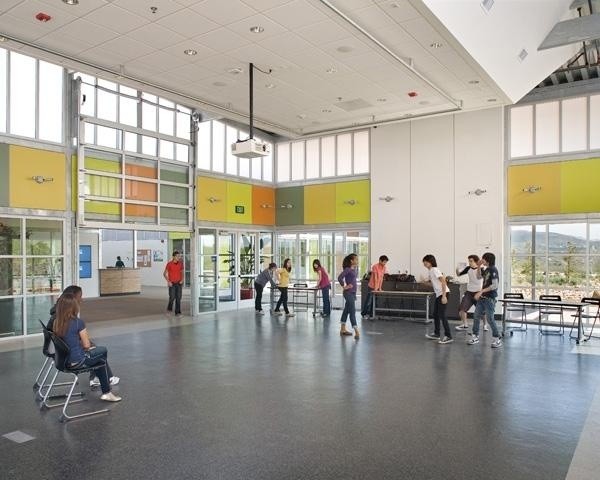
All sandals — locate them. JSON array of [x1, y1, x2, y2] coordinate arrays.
[[339, 330, 352, 335], [355, 332, 360, 340]]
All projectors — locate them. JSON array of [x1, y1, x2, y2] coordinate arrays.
[[232, 139, 270, 159]]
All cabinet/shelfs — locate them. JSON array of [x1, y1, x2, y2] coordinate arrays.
[[97, 266, 142, 296], [358, 277, 468, 322]]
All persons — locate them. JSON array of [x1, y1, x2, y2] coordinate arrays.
[[253, 261, 276, 315], [162, 251, 185, 320], [466, 251, 505, 348], [114, 256, 125, 267], [422, 255, 454, 344], [337, 253, 361, 337], [53, 292, 125, 403], [453, 253, 490, 333], [360, 254, 389, 322], [45, 285, 100, 387], [272, 258, 296, 317], [311, 260, 332, 317]]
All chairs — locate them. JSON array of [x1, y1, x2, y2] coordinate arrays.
[[394, 279, 417, 322], [537, 293, 565, 336], [569, 293, 600, 344], [291, 282, 312, 313], [38, 330, 112, 423], [28, 317, 87, 399], [499, 290, 530, 333]]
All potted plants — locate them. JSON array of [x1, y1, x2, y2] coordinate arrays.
[[219, 235, 272, 299]]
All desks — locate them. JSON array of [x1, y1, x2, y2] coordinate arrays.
[[497, 296, 590, 346], [266, 282, 332, 318], [370, 288, 439, 329]]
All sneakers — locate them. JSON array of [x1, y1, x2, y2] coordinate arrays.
[[425, 334, 440, 340], [490, 338, 502, 348], [164, 310, 171, 317], [259, 310, 265, 314], [285, 313, 296, 317], [108, 376, 121, 386], [483, 325, 489, 332], [466, 336, 480, 345], [438, 335, 454, 344], [454, 323, 469, 331], [361, 311, 369, 320], [174, 314, 184, 321], [100, 392, 122, 403], [369, 316, 377, 320], [88, 376, 100, 387], [256, 309, 259, 313], [274, 311, 282, 314]]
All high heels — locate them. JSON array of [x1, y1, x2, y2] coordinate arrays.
[[322, 312, 330, 318]]
